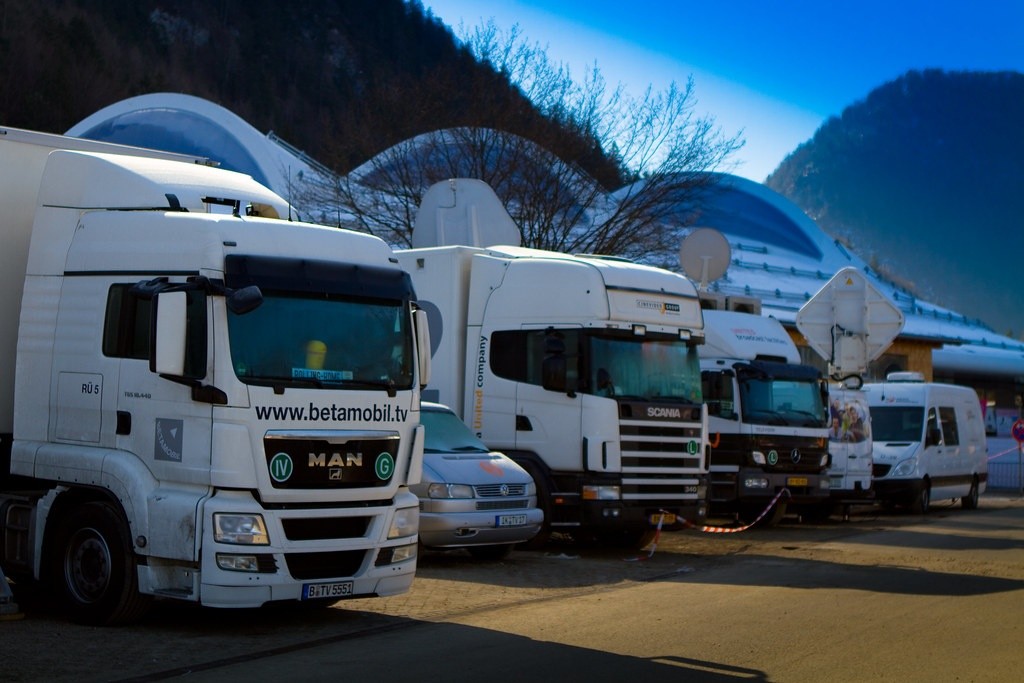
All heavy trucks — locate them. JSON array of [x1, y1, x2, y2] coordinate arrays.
[[1, 126, 431, 622], [393, 244, 721, 560]]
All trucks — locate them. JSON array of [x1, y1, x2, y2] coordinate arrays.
[[643, 310, 836, 531]]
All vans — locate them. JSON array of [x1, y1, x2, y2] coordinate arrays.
[[861, 372, 989, 516], [773, 379, 875, 525]]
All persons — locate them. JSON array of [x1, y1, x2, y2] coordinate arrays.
[[829, 396, 869, 444]]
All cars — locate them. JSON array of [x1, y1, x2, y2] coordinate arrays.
[[412, 398, 545, 553]]
